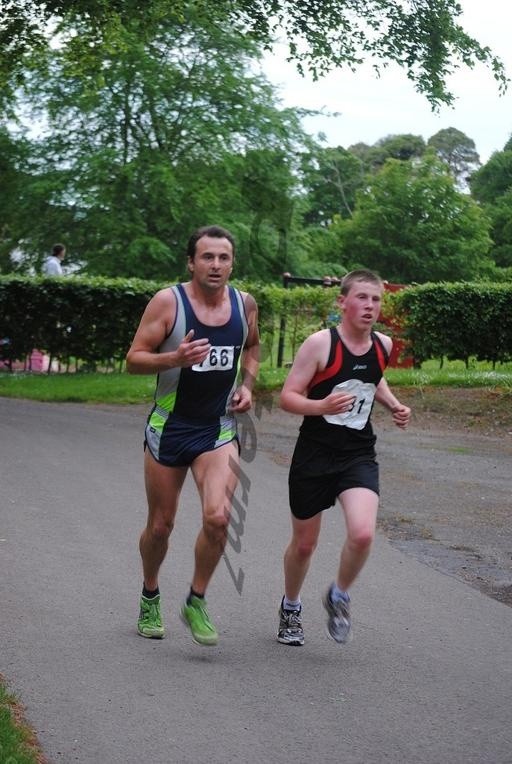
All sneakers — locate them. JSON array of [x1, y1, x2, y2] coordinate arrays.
[[182, 591, 217, 645], [138, 593, 164, 639], [279, 593, 305, 648], [323, 586, 350, 641]]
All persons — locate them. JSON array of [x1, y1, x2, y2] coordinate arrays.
[[40, 244, 65, 275], [278, 270, 412, 646], [125, 225, 260, 645]]
